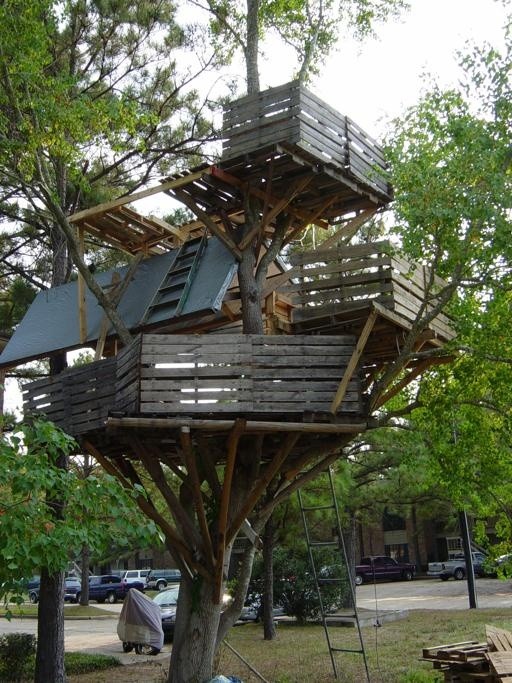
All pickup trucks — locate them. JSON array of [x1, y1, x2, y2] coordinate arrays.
[[355, 556, 417, 585], [427, 552, 488, 581]]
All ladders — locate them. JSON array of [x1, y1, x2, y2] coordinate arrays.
[[295, 465, 370, 683]]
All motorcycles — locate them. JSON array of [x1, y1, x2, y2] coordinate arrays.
[[121, 642, 160, 654]]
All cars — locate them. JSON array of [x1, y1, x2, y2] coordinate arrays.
[[28, 564, 283, 637], [485, 553, 512, 579]]
